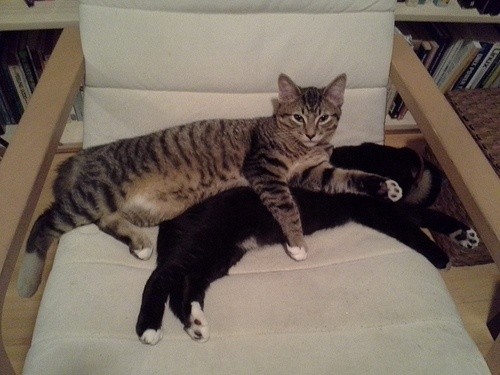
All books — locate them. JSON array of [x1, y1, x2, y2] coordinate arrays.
[[397, 0, 500, 17], [388, 28, 500, 126], [0, 32, 86, 133]]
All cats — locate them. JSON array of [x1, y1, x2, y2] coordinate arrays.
[[14, 71, 402, 299], [135, 141, 479, 346]]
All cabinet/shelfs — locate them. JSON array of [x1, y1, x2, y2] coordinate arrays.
[[0, 14, 500, 153]]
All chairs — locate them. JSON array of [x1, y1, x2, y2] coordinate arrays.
[[0, 0, 500, 374]]
